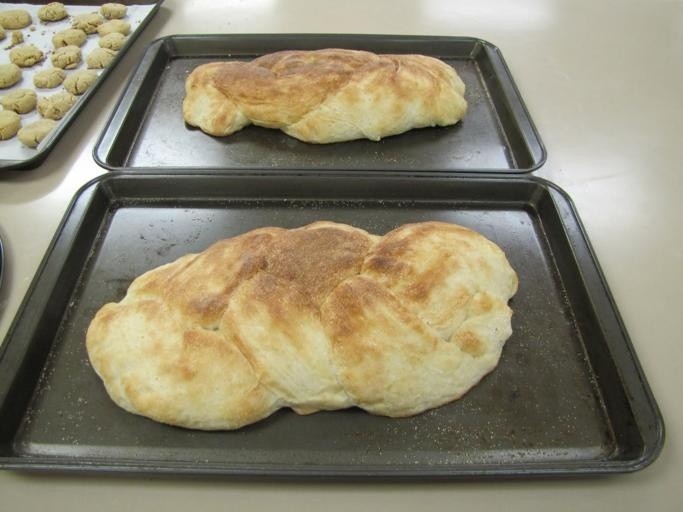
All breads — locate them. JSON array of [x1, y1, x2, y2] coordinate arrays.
[[84, 220, 518, 430], [182, 47, 469, 146]]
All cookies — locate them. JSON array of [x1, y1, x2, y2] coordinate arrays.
[[1, 2, 131, 148]]
